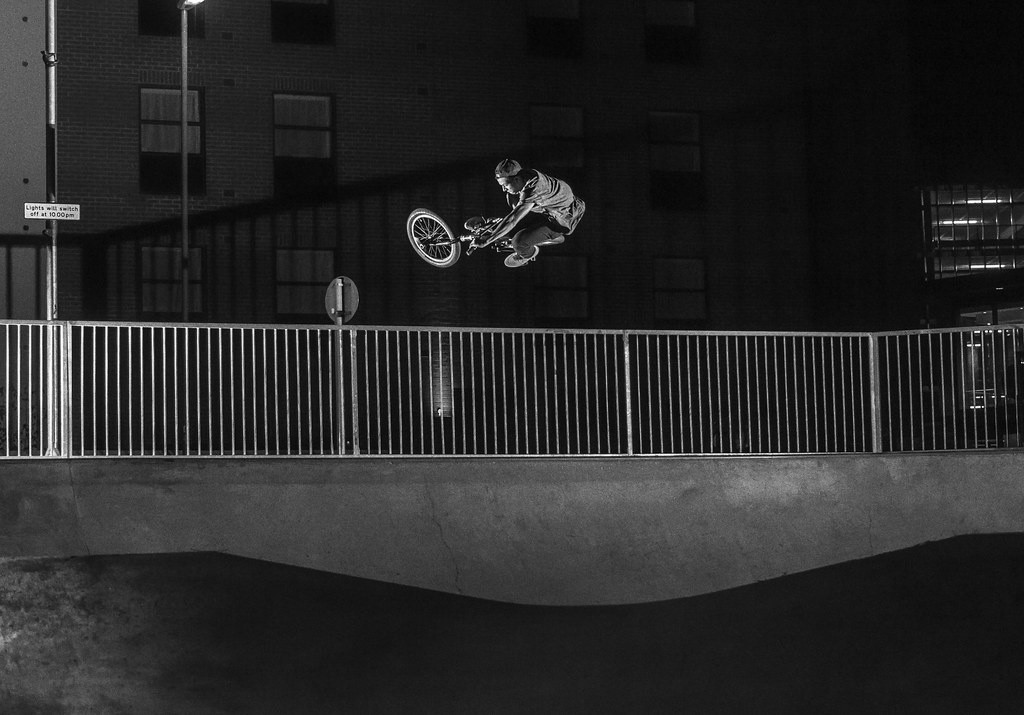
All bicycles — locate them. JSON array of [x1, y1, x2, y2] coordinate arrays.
[[405, 210, 566, 268]]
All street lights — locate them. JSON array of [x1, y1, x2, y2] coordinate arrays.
[[176, 0, 203, 320]]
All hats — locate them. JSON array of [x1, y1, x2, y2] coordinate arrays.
[[494, 158, 521, 177]]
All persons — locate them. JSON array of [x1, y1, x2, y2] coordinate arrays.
[[461, 159, 586, 272]]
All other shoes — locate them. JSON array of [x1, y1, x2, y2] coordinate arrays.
[[504, 244, 539, 268]]
